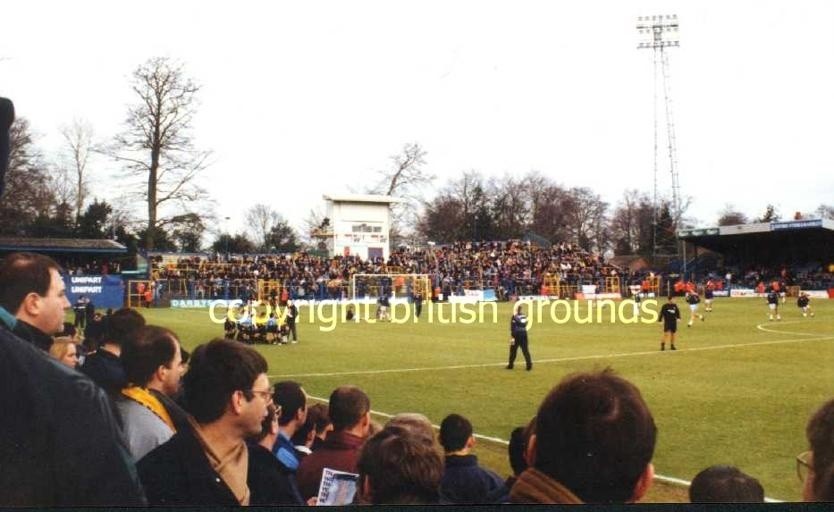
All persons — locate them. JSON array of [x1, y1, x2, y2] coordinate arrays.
[[0, 235, 833, 507]]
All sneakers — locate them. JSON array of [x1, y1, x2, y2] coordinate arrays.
[[661, 342, 677, 352]]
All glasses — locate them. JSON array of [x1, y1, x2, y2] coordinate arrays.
[[793, 447, 814, 480], [252, 384, 275, 401]]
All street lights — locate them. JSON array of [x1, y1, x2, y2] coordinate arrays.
[[637, 9, 685, 268]]
